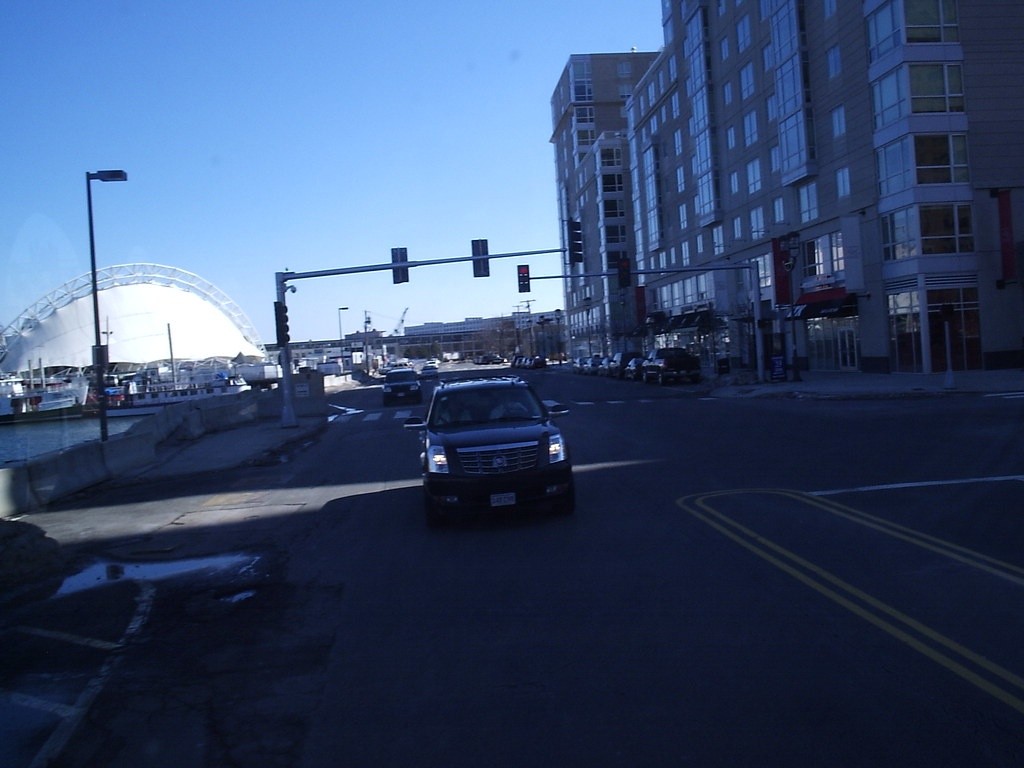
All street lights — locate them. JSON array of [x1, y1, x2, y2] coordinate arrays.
[[83, 169, 129, 440], [338, 306, 349, 374]]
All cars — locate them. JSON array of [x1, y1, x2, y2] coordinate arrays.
[[597, 355, 612, 377], [511, 354, 546, 369], [623, 356, 643, 380], [424, 360, 439, 370], [379, 369, 424, 406], [582, 357, 604, 376], [572, 356, 587, 375]]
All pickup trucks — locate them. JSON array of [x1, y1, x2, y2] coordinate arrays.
[[640, 347, 701, 386]]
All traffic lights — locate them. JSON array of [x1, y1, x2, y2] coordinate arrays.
[[567, 216, 584, 265], [516, 264, 531, 294], [616, 257, 632, 287], [274, 301, 290, 347]]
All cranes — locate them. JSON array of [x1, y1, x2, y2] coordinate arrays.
[[390, 307, 411, 337]]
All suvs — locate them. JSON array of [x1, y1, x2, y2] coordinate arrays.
[[609, 352, 638, 379], [401, 374, 578, 527]]
